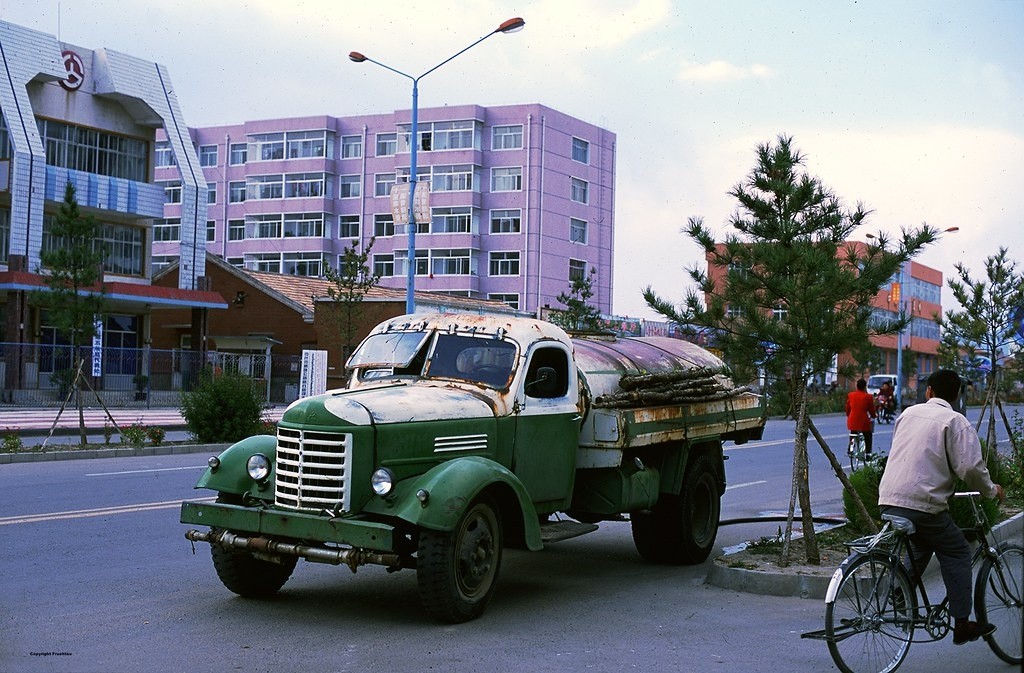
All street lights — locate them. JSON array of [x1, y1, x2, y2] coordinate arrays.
[[348, 18, 526, 310], [865, 226, 960, 415]]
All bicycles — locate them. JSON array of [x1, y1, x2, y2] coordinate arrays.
[[847, 415, 876, 472], [801, 488, 1023, 673]]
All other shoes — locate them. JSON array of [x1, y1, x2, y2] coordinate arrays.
[[883, 415, 886, 420], [866, 455, 872, 461], [890, 411, 896, 415], [889, 592, 925, 624], [952, 621, 997, 645], [847, 452, 854, 457]]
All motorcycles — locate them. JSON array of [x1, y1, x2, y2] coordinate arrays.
[[872, 393, 895, 425]]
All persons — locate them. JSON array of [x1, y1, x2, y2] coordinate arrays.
[[846, 378, 879, 461], [809, 379, 819, 394], [877, 380, 897, 420], [878, 369, 1005, 644]]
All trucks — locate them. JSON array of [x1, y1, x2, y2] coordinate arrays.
[[179, 303, 768, 625], [866, 374, 911, 407]]
